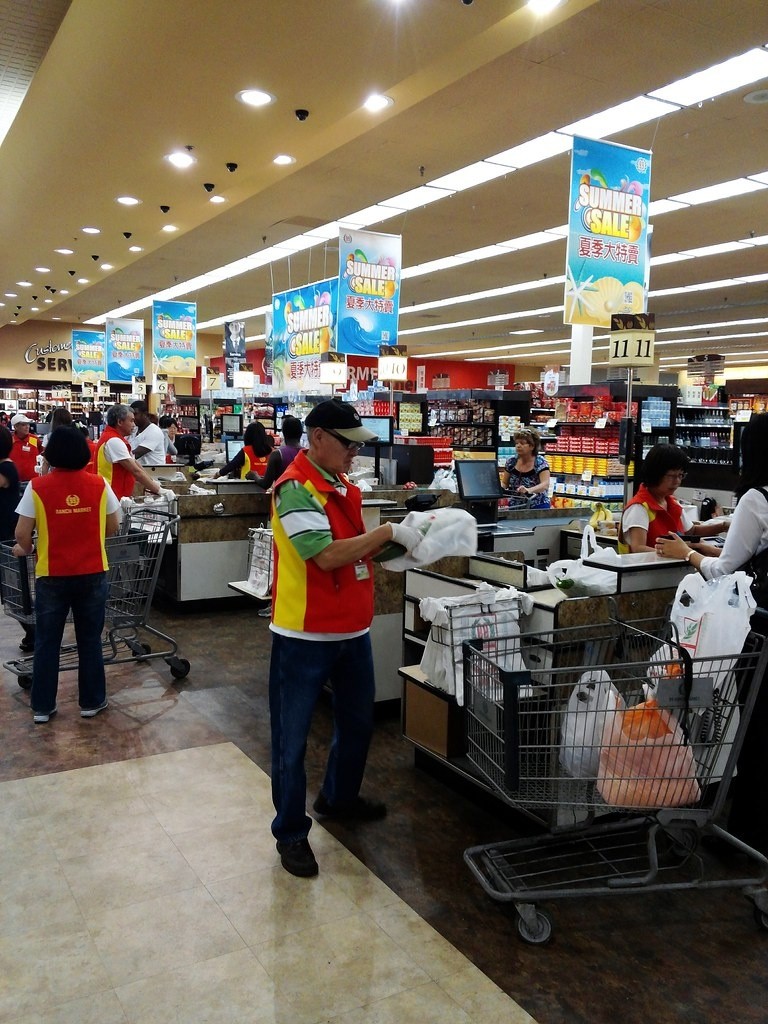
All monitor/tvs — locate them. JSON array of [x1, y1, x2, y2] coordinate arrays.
[[178, 415, 198, 432], [225, 439, 246, 463], [358, 416, 394, 447], [455, 459, 503, 499], [87, 426, 95, 441], [220, 414, 243, 435], [175, 434, 201, 455], [36, 423, 51, 434]]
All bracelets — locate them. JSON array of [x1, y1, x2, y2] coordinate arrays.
[[723, 521, 729, 533]]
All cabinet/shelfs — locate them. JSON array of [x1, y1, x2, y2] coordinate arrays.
[[531, 383, 638, 514], [38, 387, 71, 433], [0, 387, 38, 427], [642, 387, 739, 470], [71, 384, 146, 426], [423, 392, 497, 462], [157, 396, 200, 446]]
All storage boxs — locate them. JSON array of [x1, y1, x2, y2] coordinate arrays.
[[398, 666, 459, 759]]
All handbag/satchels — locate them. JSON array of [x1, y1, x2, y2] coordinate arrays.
[[559, 672, 628, 780], [596, 704, 701, 806], [735, 548, 768, 613], [650, 572, 756, 715]]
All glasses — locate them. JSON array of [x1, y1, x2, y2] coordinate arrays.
[[518, 428, 531, 432], [321, 427, 363, 450]]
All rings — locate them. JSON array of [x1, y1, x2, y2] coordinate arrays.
[[660, 550, 663, 554]]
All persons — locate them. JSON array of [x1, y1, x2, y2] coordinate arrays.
[[655, 412, 768, 870], [0, 401, 306, 617], [501, 426, 551, 510], [12, 424, 121, 722], [268, 397, 426, 877], [618, 443, 731, 555]]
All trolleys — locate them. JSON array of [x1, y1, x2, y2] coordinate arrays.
[[461, 596, 767, 945], [498, 489, 538, 510], [0, 507, 190, 689]]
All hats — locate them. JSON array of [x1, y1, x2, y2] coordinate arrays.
[[304, 398, 378, 443], [11, 414, 35, 425]]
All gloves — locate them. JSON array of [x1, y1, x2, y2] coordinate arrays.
[[387, 521, 425, 553]]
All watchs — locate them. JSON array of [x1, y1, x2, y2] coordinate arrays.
[[685, 550, 696, 562]]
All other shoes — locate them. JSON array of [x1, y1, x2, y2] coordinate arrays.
[[258, 603, 272, 617], [34, 704, 58, 721], [81, 698, 108, 716]]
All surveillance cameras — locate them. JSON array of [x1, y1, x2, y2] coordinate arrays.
[[16, 306, 22, 309], [44, 286, 51, 290], [92, 255, 99, 261], [123, 232, 132, 239], [32, 296, 38, 300], [295, 109, 309, 121], [68, 271, 75, 276], [50, 289, 56, 294], [160, 206, 170, 214], [226, 163, 238, 173], [203, 183, 215, 193], [14, 313, 19, 316]]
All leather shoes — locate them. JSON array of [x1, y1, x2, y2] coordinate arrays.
[[313, 790, 386, 819], [276, 838, 318, 877]]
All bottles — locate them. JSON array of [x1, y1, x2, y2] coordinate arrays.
[[731, 401, 749, 411], [675, 430, 730, 446], [71, 403, 83, 413], [276, 405, 288, 412], [675, 410, 733, 424], [643, 445, 654, 460], [643, 436, 657, 444], [41, 413, 45, 419], [88, 423, 94, 440], [658, 436, 669, 445]]
